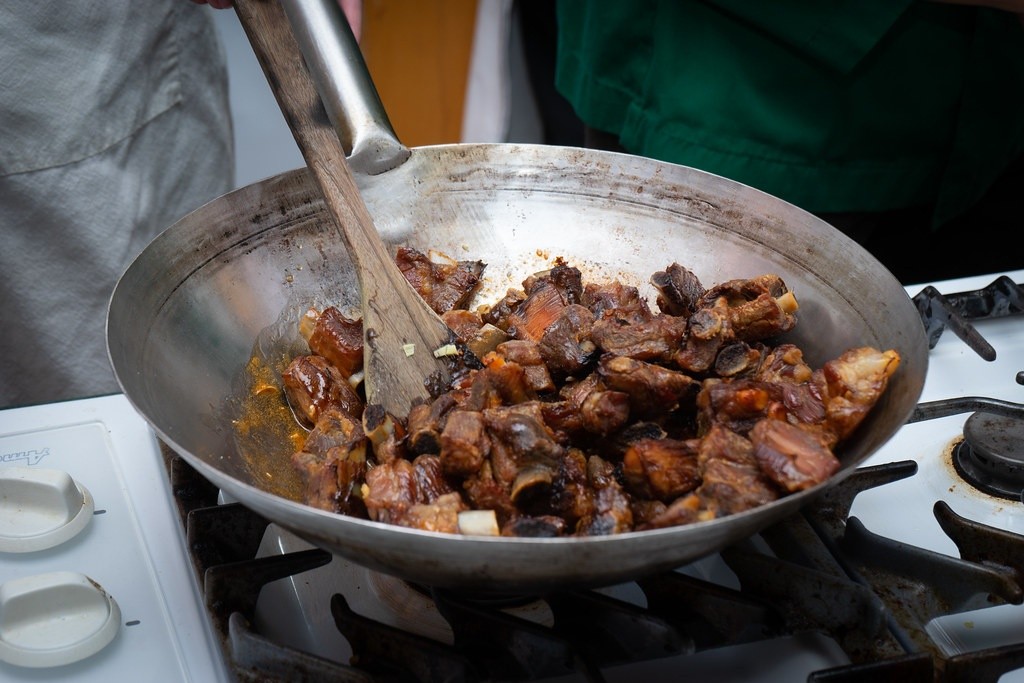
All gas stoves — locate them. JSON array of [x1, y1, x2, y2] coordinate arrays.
[[2, 263, 1024, 683]]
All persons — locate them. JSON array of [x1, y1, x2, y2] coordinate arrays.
[[0, 1, 366, 410], [555, 3, 1024, 294]]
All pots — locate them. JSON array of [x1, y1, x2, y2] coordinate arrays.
[[105, 1, 931, 595]]
